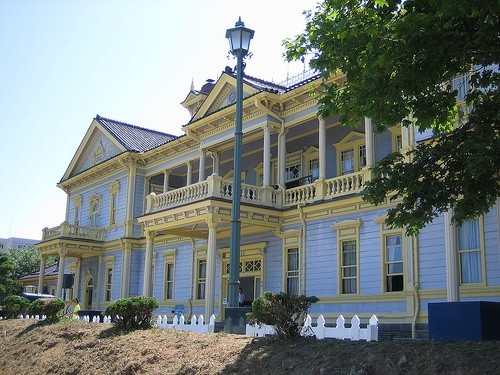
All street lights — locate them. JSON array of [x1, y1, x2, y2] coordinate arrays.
[[224, 15, 257, 308]]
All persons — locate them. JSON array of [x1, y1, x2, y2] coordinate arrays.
[[239, 287, 246, 307], [72, 297, 81, 319]]
